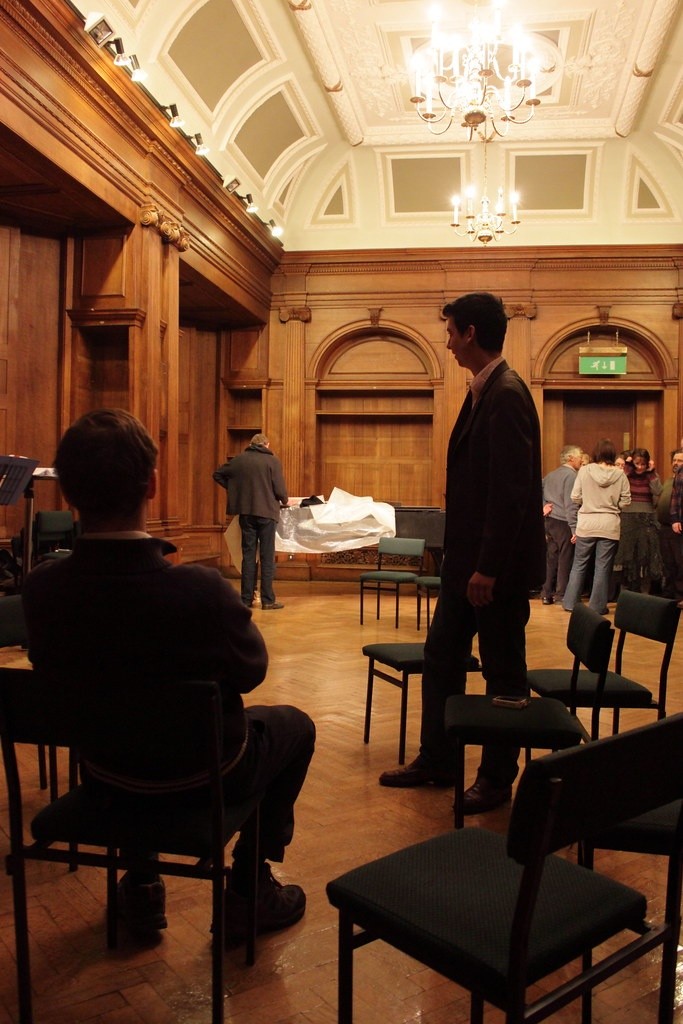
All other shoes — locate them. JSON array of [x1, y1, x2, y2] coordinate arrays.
[[262, 602, 284, 610], [542, 595, 563, 605], [602, 608, 609, 615]]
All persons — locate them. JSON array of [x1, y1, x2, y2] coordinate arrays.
[[380, 291, 545, 814], [23, 410, 316, 940], [212, 434, 296, 609], [541, 439, 683, 615]]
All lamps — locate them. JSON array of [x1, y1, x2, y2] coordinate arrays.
[[128, 54, 148, 82], [162, 103, 186, 128], [410, 0, 542, 144], [188, 132, 211, 155], [222, 173, 242, 195], [106, 36, 131, 67], [240, 193, 259, 215], [262, 219, 284, 236], [84, 12, 115, 48], [449, 117, 522, 246]]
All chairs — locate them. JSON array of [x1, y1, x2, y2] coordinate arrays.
[[576, 798, 683, 872], [525, 588, 683, 761], [357, 537, 425, 629], [361, 639, 484, 764], [328, 710, 683, 1024], [0, 664, 271, 1024], [443, 602, 616, 829], [414, 575, 441, 632]]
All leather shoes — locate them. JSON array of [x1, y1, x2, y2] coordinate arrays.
[[117, 873, 167, 935], [379, 753, 458, 789], [458, 780, 513, 813], [209, 862, 305, 940]]
